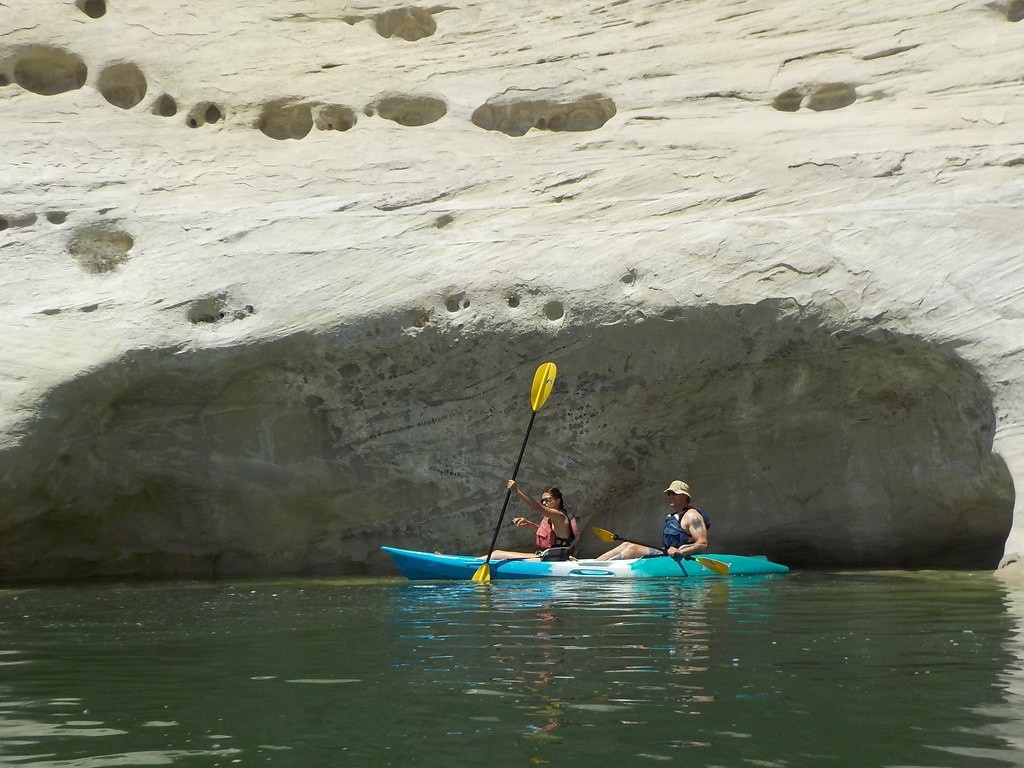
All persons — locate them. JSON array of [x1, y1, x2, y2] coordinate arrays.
[[569, 481, 709, 561], [435, 480, 575, 563]]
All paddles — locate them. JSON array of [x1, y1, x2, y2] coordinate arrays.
[[592, 525, 729, 577], [470, 362, 558, 581]]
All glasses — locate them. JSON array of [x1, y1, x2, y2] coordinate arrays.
[[666, 491, 678, 496], [540, 497, 559, 505]]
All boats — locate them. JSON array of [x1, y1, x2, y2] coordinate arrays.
[[379, 536, 791, 582]]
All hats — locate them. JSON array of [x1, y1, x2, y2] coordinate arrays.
[[663, 480, 693, 499]]
